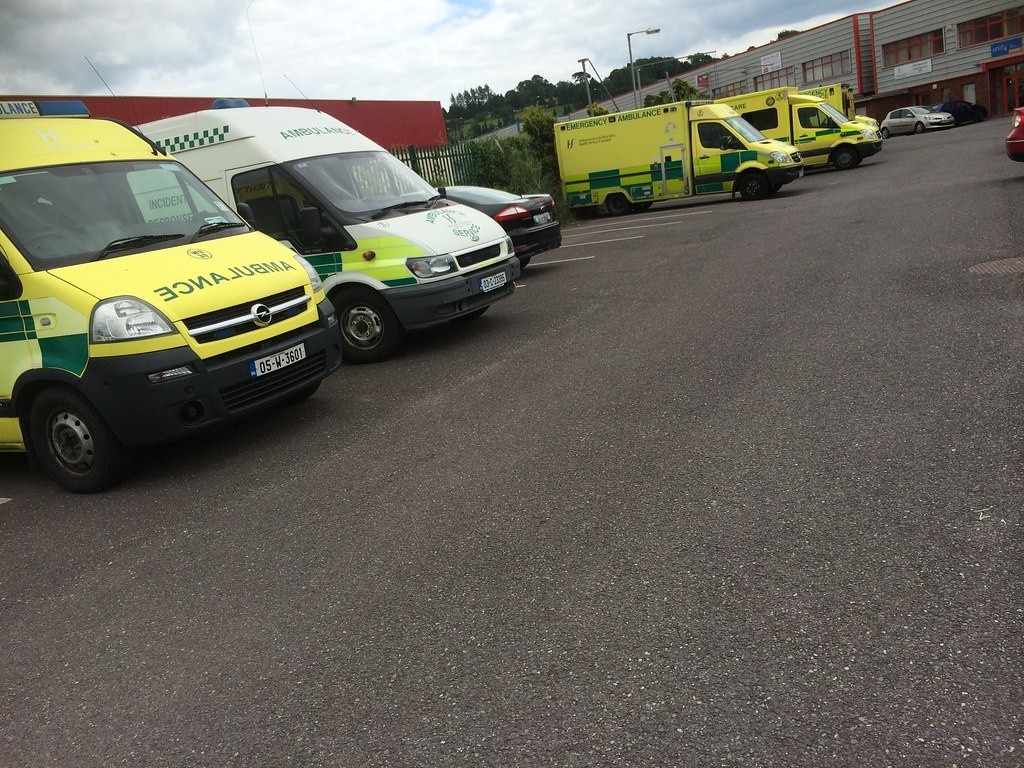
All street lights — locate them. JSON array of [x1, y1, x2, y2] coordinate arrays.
[[577, 57, 597, 117], [626, 28, 661, 109]]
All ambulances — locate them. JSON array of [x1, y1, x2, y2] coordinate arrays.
[[707, 86, 883, 170], [0, 101, 344, 497], [124, 99, 522, 362], [797, 83, 880, 130], [553, 100, 805, 215]]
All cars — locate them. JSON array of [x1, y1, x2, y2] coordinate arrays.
[[879, 106, 955, 138], [1005, 107, 1024, 162], [394, 185, 563, 270], [931, 99, 989, 126]]
